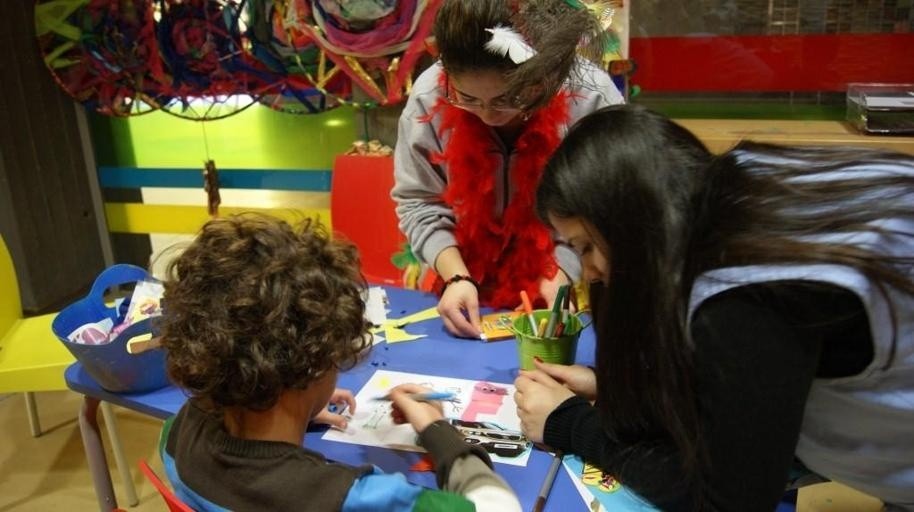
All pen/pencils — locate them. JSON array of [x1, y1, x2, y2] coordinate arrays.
[[531, 448, 564, 512], [520, 285, 571, 338], [373, 392, 452, 401]]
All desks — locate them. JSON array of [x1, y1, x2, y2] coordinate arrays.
[[669, 114, 914, 169], [62, 280, 798, 512]]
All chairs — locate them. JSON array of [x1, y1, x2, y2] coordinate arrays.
[[134, 459, 191, 512], [0, 235, 143, 511]]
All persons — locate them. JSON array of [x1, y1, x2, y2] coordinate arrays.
[[513, 103, 914, 511], [388, 0, 631, 342], [155, 206, 525, 512]]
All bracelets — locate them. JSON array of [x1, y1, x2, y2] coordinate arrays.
[[438, 272, 483, 293]]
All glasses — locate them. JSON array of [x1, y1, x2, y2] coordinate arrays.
[[444, 70, 525, 114]]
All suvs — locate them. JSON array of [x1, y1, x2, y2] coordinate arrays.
[[417, 420, 532, 460]]
